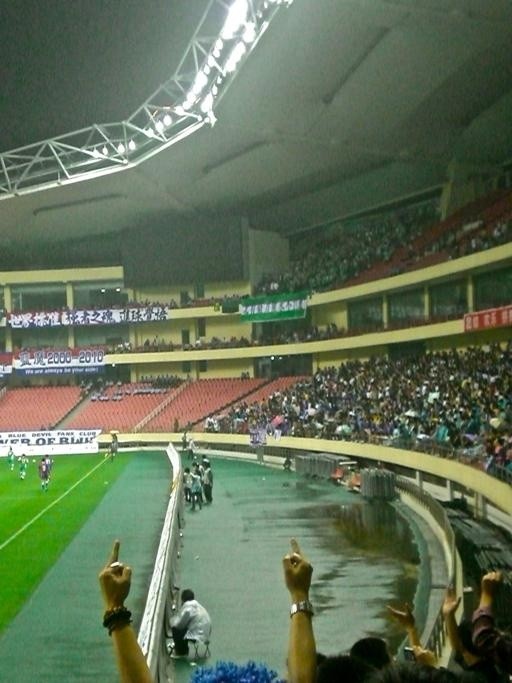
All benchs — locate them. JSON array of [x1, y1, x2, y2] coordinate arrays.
[[419, 486, 512, 682]]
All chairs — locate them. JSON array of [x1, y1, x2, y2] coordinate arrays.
[[330, 181, 512, 292], [0, 375, 313, 437]]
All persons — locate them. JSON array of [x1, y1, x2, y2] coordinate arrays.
[[0, 181, 512, 682]]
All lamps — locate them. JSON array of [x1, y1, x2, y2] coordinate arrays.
[[81, 110, 182, 159]]
